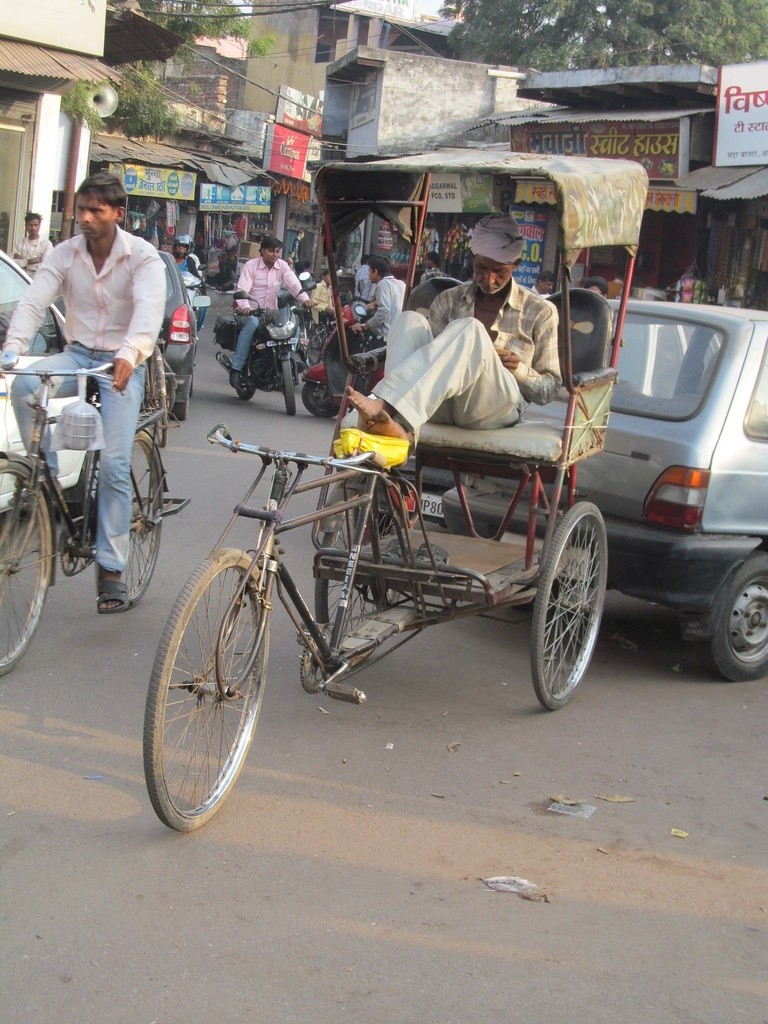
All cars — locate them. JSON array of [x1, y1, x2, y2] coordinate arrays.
[[0, 249, 88, 514], [53, 250, 211, 421]]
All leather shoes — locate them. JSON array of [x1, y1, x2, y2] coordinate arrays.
[[230, 368, 242, 390]]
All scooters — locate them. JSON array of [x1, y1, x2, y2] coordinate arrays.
[[180, 265, 208, 320], [300, 305, 384, 418]]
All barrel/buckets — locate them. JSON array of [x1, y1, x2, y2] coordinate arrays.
[[62, 413, 96, 450]]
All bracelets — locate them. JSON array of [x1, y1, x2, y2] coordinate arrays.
[[361, 323, 365, 331]]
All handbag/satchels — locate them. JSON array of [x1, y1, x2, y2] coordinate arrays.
[[51, 369, 105, 450]]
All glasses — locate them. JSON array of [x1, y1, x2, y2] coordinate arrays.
[[177, 243, 187, 248]]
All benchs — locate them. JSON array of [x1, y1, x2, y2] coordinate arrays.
[[339, 276, 611, 465]]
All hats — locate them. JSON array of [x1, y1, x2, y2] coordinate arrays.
[[468, 213, 524, 262]]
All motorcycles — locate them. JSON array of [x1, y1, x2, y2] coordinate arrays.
[[212, 281, 317, 415]]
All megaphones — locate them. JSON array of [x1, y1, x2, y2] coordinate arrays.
[[88, 85, 119, 117]]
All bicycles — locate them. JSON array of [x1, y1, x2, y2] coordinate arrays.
[[305, 312, 335, 365], [0, 348, 192, 676]]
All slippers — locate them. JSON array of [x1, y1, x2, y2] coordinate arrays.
[[8, 491, 30, 512], [385, 538, 409, 558], [97, 576, 129, 614], [416, 543, 449, 567]]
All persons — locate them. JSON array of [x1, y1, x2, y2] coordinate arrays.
[[420, 252, 451, 285], [13, 213, 54, 280], [173, 233, 207, 341], [531, 271, 556, 298], [345, 212, 563, 468], [583, 276, 608, 295], [132, 228, 144, 238], [229, 237, 317, 389], [286, 250, 295, 273], [293, 230, 304, 276], [355, 255, 374, 317], [351, 256, 407, 393], [310, 268, 335, 317], [0, 170, 167, 614]]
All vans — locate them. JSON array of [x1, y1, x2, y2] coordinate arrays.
[[443, 299, 768, 682]]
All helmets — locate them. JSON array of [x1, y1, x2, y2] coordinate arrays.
[[173, 236, 190, 256], [182, 234, 195, 252]]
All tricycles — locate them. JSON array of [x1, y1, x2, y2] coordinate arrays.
[[142, 149, 648, 833]]
